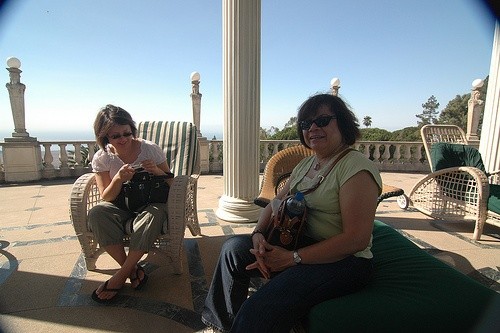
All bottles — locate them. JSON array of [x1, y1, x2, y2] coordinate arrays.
[[122, 178, 135, 193], [285, 191, 306, 216]]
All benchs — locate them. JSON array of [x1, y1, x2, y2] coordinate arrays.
[[246, 218, 500, 333]]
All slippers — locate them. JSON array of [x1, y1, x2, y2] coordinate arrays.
[[91, 280, 122, 304], [130, 264, 145, 290]]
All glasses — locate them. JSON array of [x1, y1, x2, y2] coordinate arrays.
[[300, 115, 339, 130], [105, 133, 132, 139]]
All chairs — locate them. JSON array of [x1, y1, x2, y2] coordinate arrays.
[[71, 120, 202, 274], [254, 145, 404, 210], [396, 124, 500, 241]]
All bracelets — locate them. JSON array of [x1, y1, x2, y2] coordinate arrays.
[[251, 230, 263, 238]]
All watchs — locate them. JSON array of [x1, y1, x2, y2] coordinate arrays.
[[293, 250, 301, 265]]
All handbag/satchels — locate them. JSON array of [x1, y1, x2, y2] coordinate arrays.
[[118, 165, 171, 211], [262, 195, 307, 251]]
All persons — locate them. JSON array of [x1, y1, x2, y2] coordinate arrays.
[[196, 94, 383, 333], [87, 105, 173, 304]]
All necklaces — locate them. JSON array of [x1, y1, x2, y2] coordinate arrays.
[[314, 145, 343, 170]]
